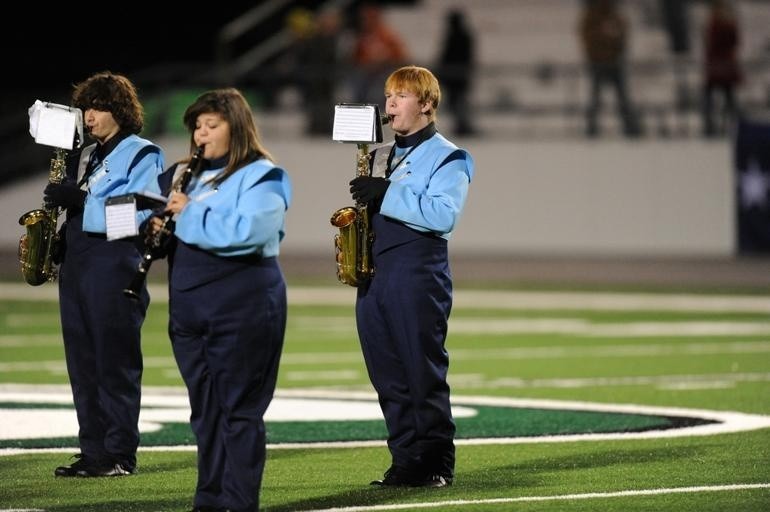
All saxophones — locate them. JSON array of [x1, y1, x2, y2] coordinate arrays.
[[330, 114, 396, 286], [19, 126, 93, 286]]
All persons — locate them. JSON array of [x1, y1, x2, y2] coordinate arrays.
[[351, 5, 403, 114], [580, 1, 640, 137], [40, 70, 163, 480], [126, 86, 295, 512], [432, 7, 477, 134], [294, 3, 340, 135], [643, 1, 743, 136], [350, 64, 476, 488]]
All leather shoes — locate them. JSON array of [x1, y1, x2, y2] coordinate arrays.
[[51, 455, 137, 480], [371, 467, 455, 491]]
